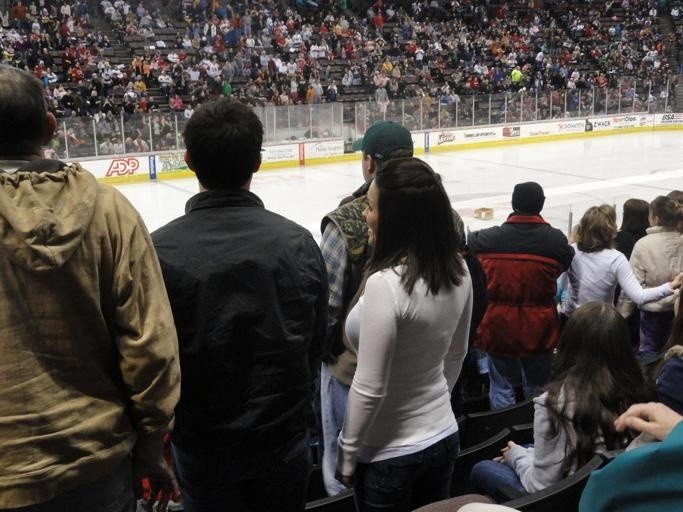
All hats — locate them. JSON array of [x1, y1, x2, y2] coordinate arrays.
[[352, 121, 413, 161], [511, 182, 545, 215]]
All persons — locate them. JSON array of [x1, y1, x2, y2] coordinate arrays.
[[463, 183, 682, 512], [0, 65, 184, 512], [318, 120, 467, 497], [334, 157, 474, 511], [149, 96, 329, 512], [2, 0, 683, 159]]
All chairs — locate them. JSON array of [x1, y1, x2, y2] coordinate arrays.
[[304, 487, 359, 512], [445, 394, 630, 512]]
